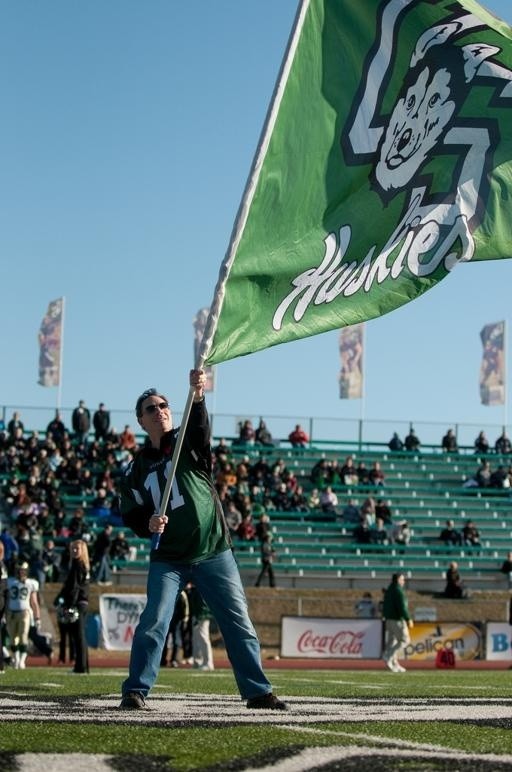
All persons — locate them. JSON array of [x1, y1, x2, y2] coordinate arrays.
[[0, 399, 136, 603], [210, 418, 410, 588], [1, 541, 15, 674], [440, 518, 511, 599], [444, 427, 512, 488], [160, 582, 217, 671], [378, 573, 414, 674], [388, 427, 421, 458], [7, 562, 42, 670], [122, 370, 284, 711], [55, 540, 92, 672]]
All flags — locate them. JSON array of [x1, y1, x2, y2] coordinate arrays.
[[37, 299, 65, 389], [478, 318, 506, 407], [192, 305, 215, 395], [201, 2, 510, 363], [336, 322, 365, 400]]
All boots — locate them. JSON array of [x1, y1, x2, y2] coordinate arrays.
[[13, 650, 20, 670], [20, 651, 28, 669]]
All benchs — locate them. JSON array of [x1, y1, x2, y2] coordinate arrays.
[[0, 443, 512, 590]]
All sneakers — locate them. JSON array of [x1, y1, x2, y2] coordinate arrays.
[[120, 691, 146, 709], [246, 692, 287, 710], [382, 654, 394, 671], [392, 663, 406, 673], [166, 656, 215, 672]]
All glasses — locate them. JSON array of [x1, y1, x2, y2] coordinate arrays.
[[140, 402, 169, 417]]
